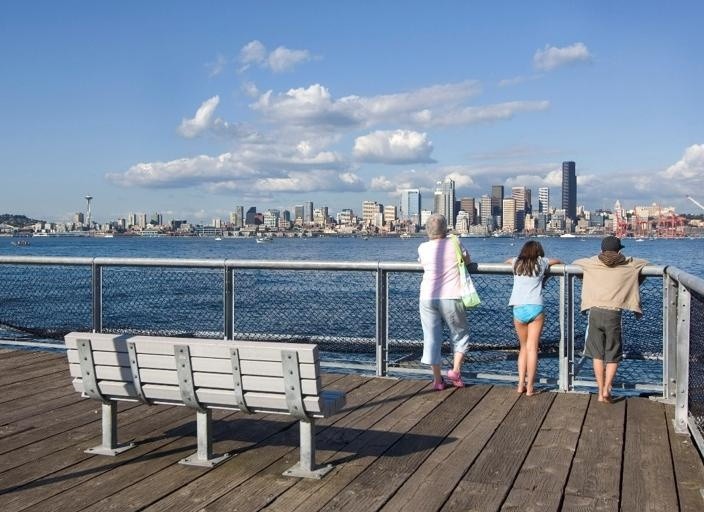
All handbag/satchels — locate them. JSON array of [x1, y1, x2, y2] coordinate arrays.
[[458, 261, 482, 308]]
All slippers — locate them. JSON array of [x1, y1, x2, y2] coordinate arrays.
[[433, 379, 445, 390], [447, 369, 464, 387]]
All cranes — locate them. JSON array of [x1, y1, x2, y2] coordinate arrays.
[[615, 205, 686, 237]]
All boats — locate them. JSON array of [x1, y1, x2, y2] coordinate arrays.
[[11, 240, 30, 248], [400, 232, 423, 240], [494, 232, 515, 237], [560, 234, 575, 237], [257, 237, 273, 243], [447, 233, 487, 238]]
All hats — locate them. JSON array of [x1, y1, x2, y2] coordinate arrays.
[[601, 236, 625, 251]]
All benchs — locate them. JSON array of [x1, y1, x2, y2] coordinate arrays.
[[63, 330, 349, 481]]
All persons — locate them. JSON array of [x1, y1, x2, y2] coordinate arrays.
[[501, 241, 563, 396], [570, 235, 655, 403], [417, 215, 483, 392]]
